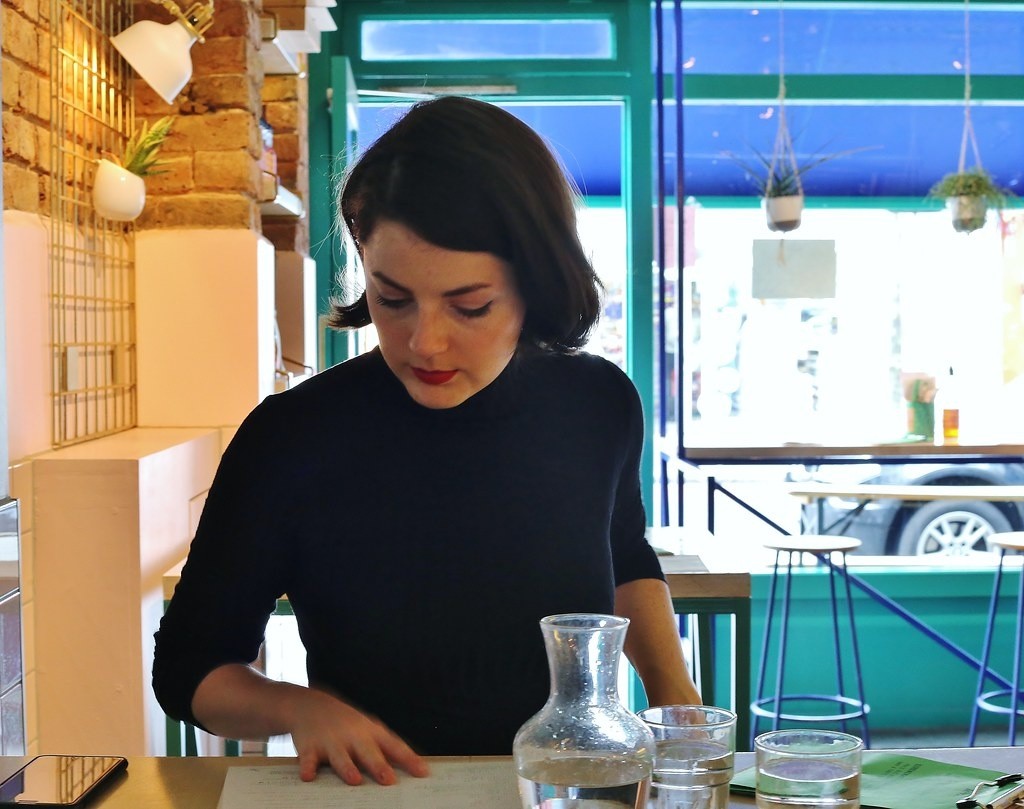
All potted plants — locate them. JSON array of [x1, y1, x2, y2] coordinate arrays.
[[718, 125, 885, 234], [923, 172, 1005, 234], [92, 114, 177, 223]]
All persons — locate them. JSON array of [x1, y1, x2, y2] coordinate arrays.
[[152, 95, 709, 785]]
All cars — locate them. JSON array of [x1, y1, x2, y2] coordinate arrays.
[[802, 375, 1024, 560]]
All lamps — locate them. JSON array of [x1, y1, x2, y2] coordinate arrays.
[[108, 0, 215, 106]]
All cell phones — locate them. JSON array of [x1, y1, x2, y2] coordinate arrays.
[[0, 754, 129, 809]]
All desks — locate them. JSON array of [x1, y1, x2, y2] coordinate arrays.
[[637, 525, 751, 752], [683, 441, 1024, 465], [0, 747, 1024, 809], [787, 484, 1024, 566], [162, 556, 293, 757]]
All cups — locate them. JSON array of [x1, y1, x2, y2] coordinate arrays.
[[636, 704, 738, 809], [751, 729, 865, 809], [943, 409, 959, 438]]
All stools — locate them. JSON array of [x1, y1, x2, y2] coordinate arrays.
[[967, 531, 1024, 747], [749, 535, 871, 753]]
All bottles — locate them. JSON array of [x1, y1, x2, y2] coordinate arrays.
[[511, 613, 656, 809]]
